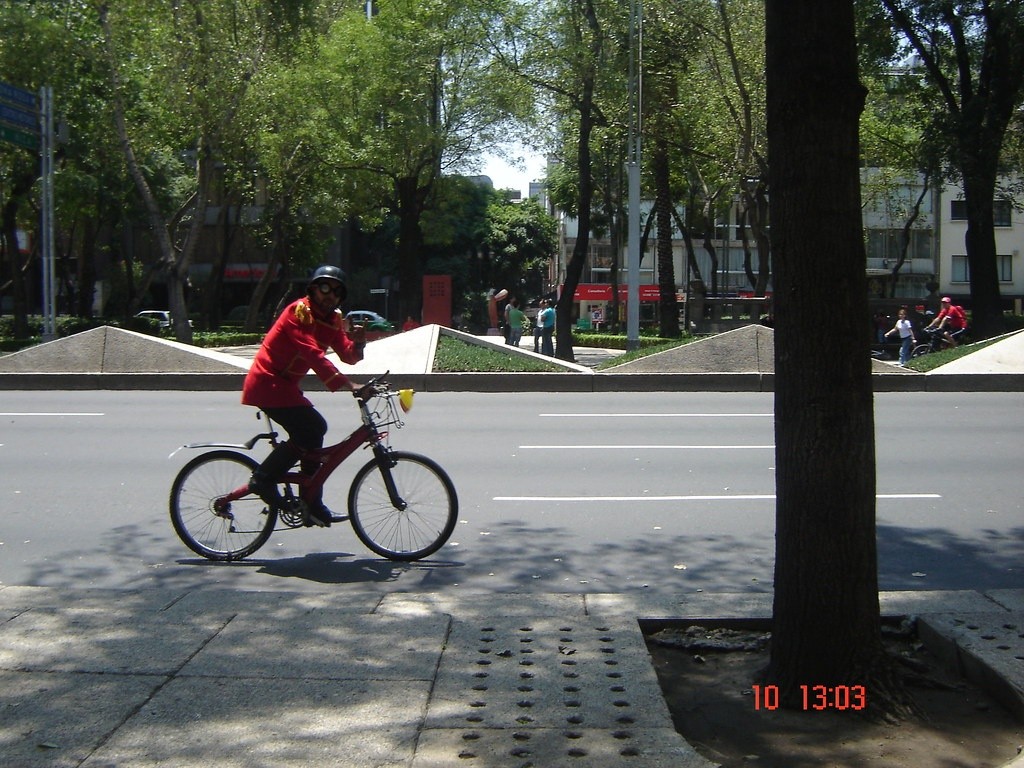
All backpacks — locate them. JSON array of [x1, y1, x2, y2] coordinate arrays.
[[949, 305, 967, 320]]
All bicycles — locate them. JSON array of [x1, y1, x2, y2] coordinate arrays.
[[911, 328, 958, 360], [167, 370, 460, 563]]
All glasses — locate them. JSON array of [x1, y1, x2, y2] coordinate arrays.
[[310, 281, 344, 296]]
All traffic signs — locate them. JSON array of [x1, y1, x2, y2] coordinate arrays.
[[0, 82, 37, 110], [0, 120, 41, 153], [0, 103, 36, 129]]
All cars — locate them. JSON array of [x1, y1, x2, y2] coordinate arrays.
[[134, 310, 195, 329], [346, 312, 389, 330]]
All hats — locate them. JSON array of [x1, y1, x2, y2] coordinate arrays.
[[941, 297, 951, 302]]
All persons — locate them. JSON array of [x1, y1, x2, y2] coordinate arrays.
[[875, 298, 967, 365], [242, 265, 368, 527], [402, 315, 421, 333], [533, 299, 555, 357], [591, 305, 603, 329], [504, 296, 526, 347]]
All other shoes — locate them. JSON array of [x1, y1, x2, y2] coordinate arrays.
[[248, 473, 282, 505], [309, 499, 331, 528]]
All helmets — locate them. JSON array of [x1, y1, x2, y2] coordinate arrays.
[[311, 265, 345, 281]]
